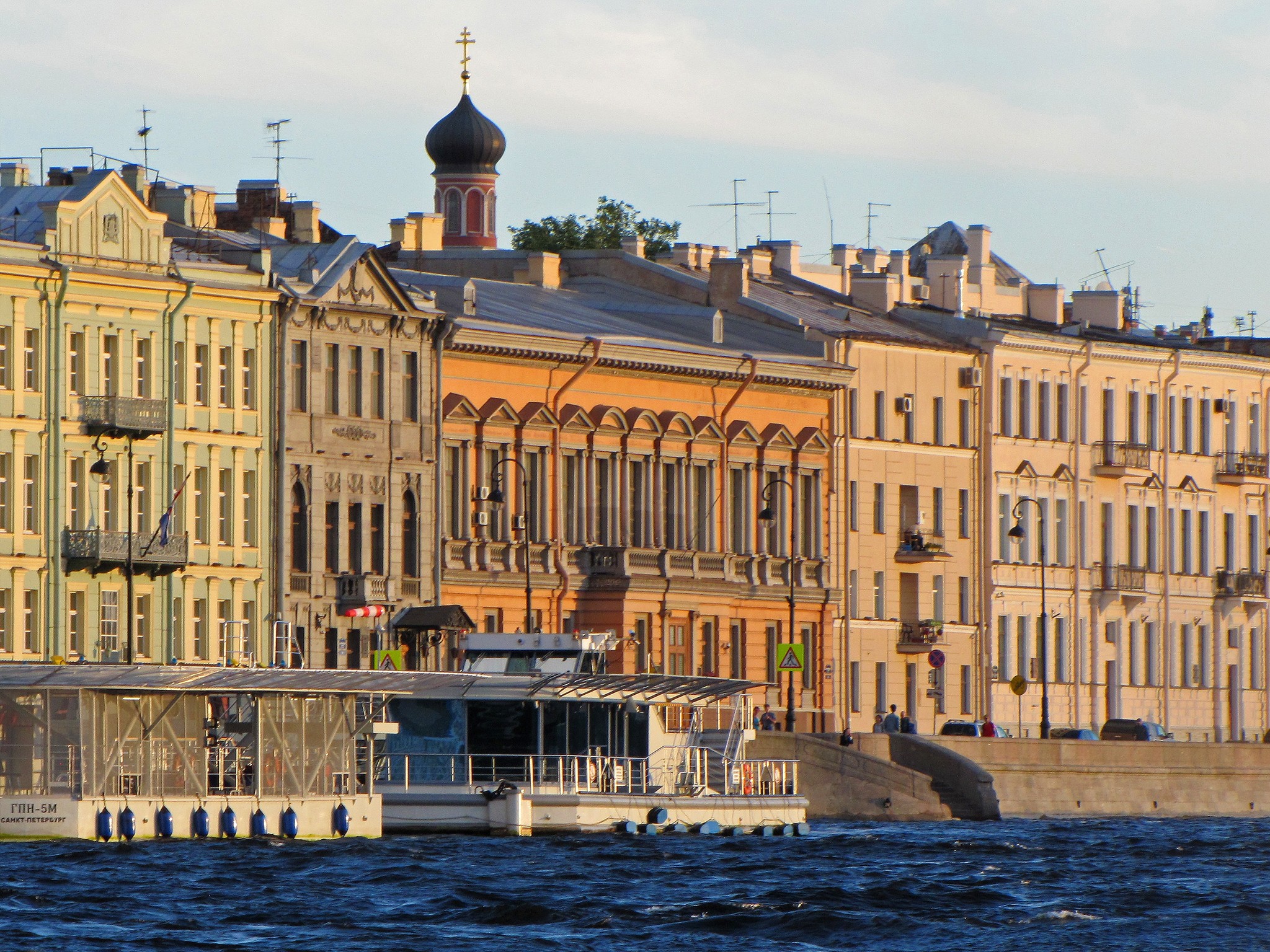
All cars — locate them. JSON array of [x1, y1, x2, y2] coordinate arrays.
[[1049, 727, 1099, 740]]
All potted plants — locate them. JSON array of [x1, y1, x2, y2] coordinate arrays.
[[926, 542, 943, 552]]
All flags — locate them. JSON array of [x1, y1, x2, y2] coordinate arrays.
[[160, 477, 189, 546]]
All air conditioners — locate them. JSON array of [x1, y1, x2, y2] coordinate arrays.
[[896, 397, 912, 412], [1215, 398, 1229, 413], [513, 516, 525, 529], [474, 487, 489, 500], [474, 512, 487, 525], [965, 367, 981, 386]]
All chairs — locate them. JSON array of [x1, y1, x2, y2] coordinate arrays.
[[902, 623, 912, 642]]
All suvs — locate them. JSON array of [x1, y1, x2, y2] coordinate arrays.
[[937, 719, 1013, 739]]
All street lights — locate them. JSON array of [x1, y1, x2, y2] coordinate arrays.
[[1006, 498, 1051, 740], [756, 479, 797, 732], [90, 425, 134, 667], [480, 458, 534, 634]]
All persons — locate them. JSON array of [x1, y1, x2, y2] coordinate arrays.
[[981, 714, 995, 737], [882, 704, 901, 733], [839, 727, 854, 747], [760, 704, 777, 731], [900, 711, 918, 734], [872, 715, 884, 733], [753, 706, 762, 730], [1134, 718, 1150, 741]]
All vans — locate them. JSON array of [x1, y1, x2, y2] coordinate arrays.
[[1099, 718, 1174, 742]]
